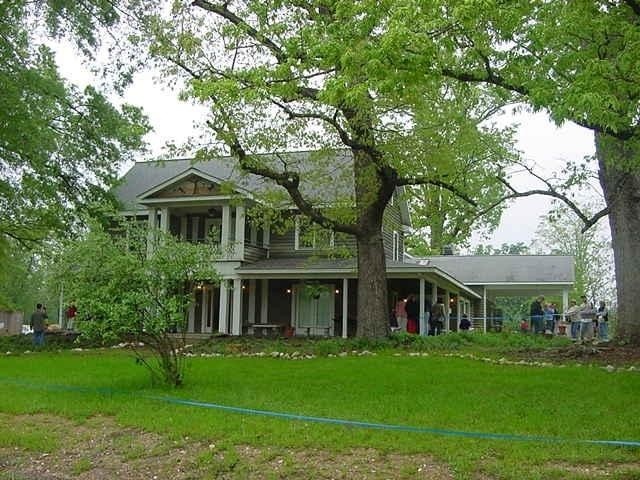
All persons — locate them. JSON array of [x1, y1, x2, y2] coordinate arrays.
[[389, 294, 446, 336], [66, 305, 76, 330], [519, 295, 609, 340], [30, 304, 47, 352], [459, 313, 472, 333]]
